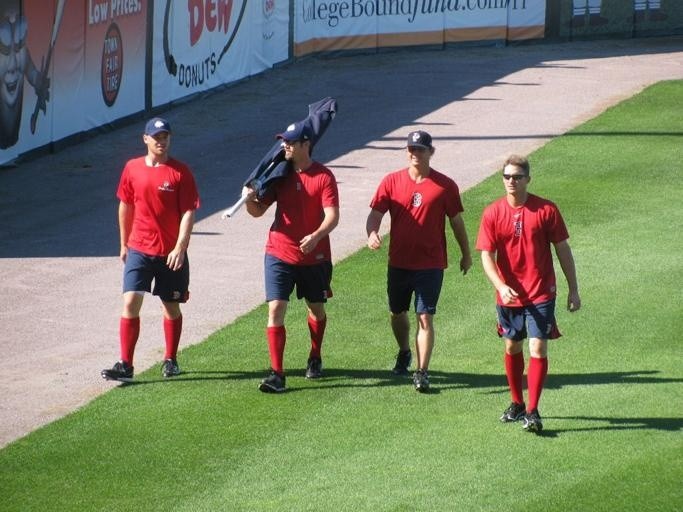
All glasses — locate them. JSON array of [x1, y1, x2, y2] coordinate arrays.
[[502, 174, 526, 180]]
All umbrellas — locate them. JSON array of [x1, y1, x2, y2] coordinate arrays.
[[220, 94, 338, 229]]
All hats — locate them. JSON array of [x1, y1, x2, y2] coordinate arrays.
[[407, 131, 431, 149], [145, 118, 169, 136], [277, 123, 311, 142]]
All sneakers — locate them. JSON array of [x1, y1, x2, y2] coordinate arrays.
[[258, 371, 285, 391], [101, 361, 134, 382], [394, 349, 411, 373], [413, 369, 430, 391], [522, 411, 542, 431], [306, 356, 321, 378], [500, 402, 526, 421], [161, 359, 180, 377]]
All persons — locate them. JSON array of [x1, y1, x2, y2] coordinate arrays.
[[100, 115, 201, 385], [474, 154, 582, 434], [363, 129, 471, 392], [242, 119, 340, 394], [0, 1, 27, 150]]
